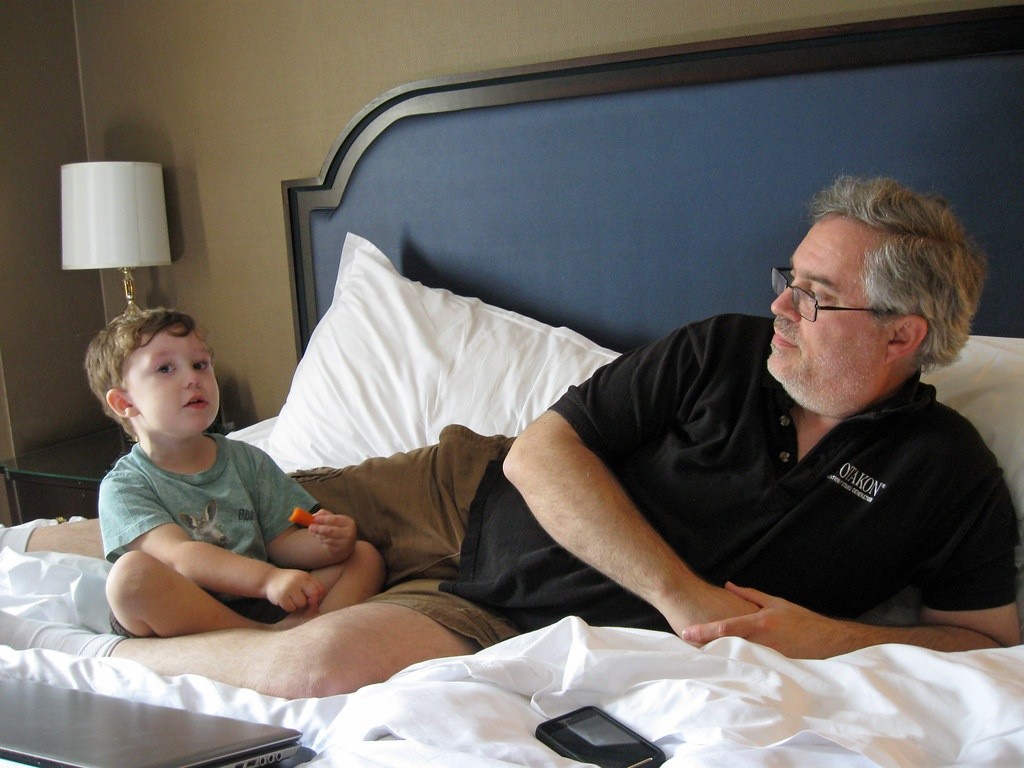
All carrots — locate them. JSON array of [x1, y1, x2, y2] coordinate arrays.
[[287, 507, 315, 527]]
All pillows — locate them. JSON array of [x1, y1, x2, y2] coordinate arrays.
[[224, 230, 624, 473], [920, 336, 1024, 569]]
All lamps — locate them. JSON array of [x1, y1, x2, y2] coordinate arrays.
[[60, 161, 172, 312]]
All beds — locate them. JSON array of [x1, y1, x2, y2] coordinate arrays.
[[0, 6, 1024, 768]]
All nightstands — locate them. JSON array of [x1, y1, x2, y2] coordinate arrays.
[[0, 427, 210, 526]]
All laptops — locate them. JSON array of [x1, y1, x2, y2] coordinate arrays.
[[0, 677, 303, 768]]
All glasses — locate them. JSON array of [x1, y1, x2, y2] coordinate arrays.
[[770, 265, 900, 322]]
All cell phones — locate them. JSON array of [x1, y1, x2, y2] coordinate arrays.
[[536, 705, 666, 768]]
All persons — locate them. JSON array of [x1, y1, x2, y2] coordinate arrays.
[[0, 174, 1024, 700], [84, 308, 385, 638]]
